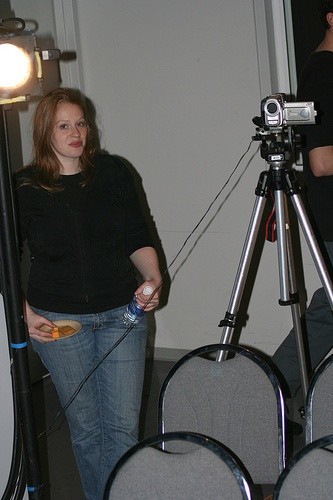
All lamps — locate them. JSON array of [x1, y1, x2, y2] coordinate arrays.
[[0, 33, 61, 108]]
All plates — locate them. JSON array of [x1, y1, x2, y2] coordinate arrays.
[[41, 319, 82, 340]]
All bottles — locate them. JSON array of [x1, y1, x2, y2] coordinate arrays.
[[122, 285, 154, 328]]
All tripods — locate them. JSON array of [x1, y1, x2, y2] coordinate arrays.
[[215, 169, 333, 411]]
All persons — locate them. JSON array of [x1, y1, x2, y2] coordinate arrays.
[[12, 88, 164, 500], [296, 0, 333, 285]]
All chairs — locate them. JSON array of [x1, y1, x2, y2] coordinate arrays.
[[103, 345, 333, 500]]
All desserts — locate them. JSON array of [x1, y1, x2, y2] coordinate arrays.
[[52, 326, 60, 339]]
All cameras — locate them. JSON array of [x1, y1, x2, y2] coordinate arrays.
[[262, 92, 319, 128]]
